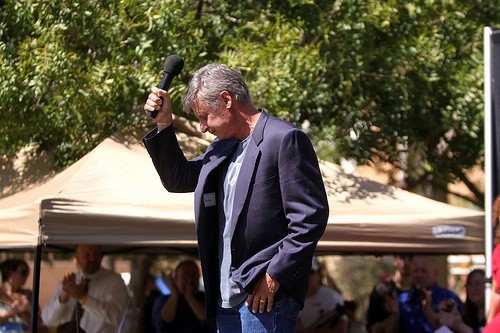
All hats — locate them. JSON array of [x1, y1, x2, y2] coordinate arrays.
[[311, 257, 320, 271]]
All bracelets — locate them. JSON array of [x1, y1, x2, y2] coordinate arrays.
[[80, 294, 88, 305]]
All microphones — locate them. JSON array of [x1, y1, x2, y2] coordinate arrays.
[[146, 55, 184, 118]]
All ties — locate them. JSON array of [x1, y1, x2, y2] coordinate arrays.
[[70, 277, 90, 325]]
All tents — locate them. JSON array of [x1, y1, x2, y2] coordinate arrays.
[[0, 136, 485, 333]]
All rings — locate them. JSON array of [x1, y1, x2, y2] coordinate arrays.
[[260, 300, 264, 303]]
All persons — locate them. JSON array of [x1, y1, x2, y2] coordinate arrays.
[[143, 64, 330, 333], [0, 198, 500, 333]]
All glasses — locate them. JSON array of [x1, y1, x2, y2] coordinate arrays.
[[397, 266, 412, 279], [465, 280, 484, 287]]
